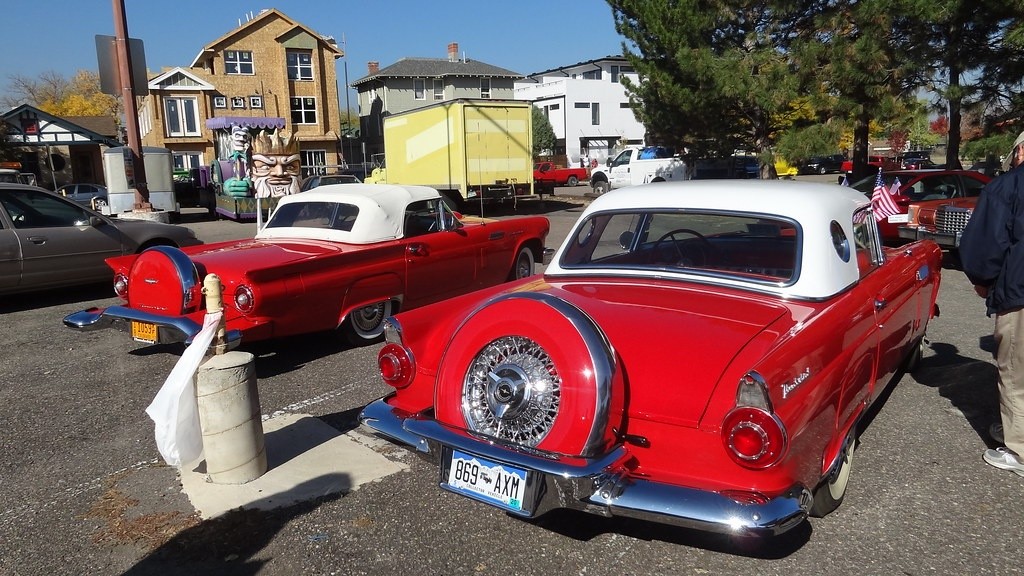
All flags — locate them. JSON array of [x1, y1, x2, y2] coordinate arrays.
[[842, 179, 849, 187], [889, 179, 901, 195], [852, 199, 868, 223], [872, 169, 902, 222]]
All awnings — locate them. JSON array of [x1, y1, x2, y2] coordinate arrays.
[[206, 117, 286, 129]]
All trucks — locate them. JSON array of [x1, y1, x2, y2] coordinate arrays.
[[363, 96, 539, 209]]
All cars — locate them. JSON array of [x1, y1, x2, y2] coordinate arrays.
[[849, 167, 994, 244], [354, 178, 943, 547], [300, 174, 362, 196], [0, 161, 37, 187], [52, 183, 108, 207], [0, 181, 204, 298], [897, 194, 980, 254], [59, 183, 556, 362], [805, 150, 936, 177]]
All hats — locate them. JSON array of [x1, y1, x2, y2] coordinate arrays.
[[1002, 131, 1024, 172]]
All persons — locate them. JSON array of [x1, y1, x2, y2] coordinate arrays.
[[583, 155, 590, 168], [958, 132, 1024, 477], [224, 128, 302, 198], [592, 159, 598, 168], [373, 159, 381, 168]]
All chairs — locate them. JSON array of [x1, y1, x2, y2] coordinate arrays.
[[922, 184, 949, 201], [734, 249, 775, 279]]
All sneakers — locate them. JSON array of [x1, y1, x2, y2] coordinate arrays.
[[983, 447, 1024, 477], [989, 422, 1004, 443]]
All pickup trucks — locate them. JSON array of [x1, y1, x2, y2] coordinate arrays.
[[533, 162, 586, 187], [587, 146, 699, 195]]
